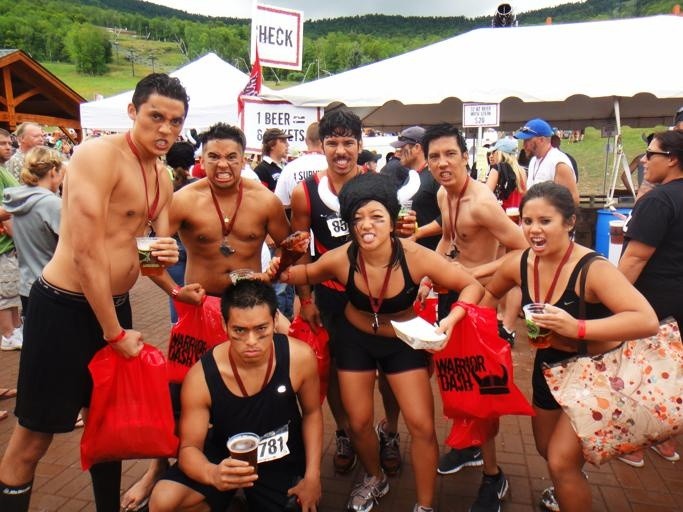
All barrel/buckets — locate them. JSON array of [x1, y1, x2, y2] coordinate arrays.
[[595, 208, 632, 257]]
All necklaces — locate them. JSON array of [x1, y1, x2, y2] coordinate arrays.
[[211, 187, 237, 223]]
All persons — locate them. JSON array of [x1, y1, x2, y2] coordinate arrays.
[[356, 149, 381, 171], [378, 148, 408, 189], [486, 146, 497, 166], [267, 172, 483, 512], [622, 133, 654, 200], [389, 125, 442, 251], [416, 121, 531, 512], [486, 136, 527, 321], [121, 123, 292, 512], [518, 140, 533, 166], [0, 72, 189, 512], [163, 142, 202, 331], [483, 181, 660, 511], [6, 122, 44, 185], [148, 277, 322, 512], [0, 128, 24, 351], [2, 144, 85, 428], [514, 119, 580, 209], [618, 130, 683, 467], [254, 128, 293, 321], [290, 111, 403, 474], [551, 136, 578, 181], [44, 130, 79, 159], [553, 127, 584, 143], [274, 122, 329, 222]]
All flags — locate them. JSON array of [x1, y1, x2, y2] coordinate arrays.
[[237, 48, 261, 116]]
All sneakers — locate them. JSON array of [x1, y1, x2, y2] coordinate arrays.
[[470, 467, 508, 512], [374, 419, 401, 474], [346, 469, 389, 512], [414, 503, 435, 511], [541, 485, 559, 512], [332, 428, 357, 471], [0, 329, 23, 351], [437, 447, 484, 474]]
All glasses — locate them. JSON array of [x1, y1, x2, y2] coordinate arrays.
[[646, 150, 670, 160]]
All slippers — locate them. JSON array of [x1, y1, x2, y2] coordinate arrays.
[[617, 457, 645, 467], [651, 445, 679, 461]]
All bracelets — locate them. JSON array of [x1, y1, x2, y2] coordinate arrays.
[[103, 327, 125, 344], [300, 297, 312, 308], [577, 319, 586, 341], [169, 286, 180, 298], [421, 281, 433, 289]]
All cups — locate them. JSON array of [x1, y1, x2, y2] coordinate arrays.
[[137, 237, 158, 268], [227, 432, 259, 475], [397, 200, 413, 237], [229, 268, 254, 283], [524, 302, 553, 338], [610, 221, 623, 244]]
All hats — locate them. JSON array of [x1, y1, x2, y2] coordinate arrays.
[[389, 125, 426, 148], [514, 118, 554, 139], [358, 150, 382, 162], [493, 136, 518, 152], [482, 128, 498, 146], [262, 128, 293, 141]]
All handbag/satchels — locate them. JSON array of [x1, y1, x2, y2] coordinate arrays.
[[168, 295, 229, 383], [541, 257, 683, 468], [80, 344, 180, 470], [434, 302, 537, 419], [493, 164, 517, 201], [289, 315, 330, 406]]
